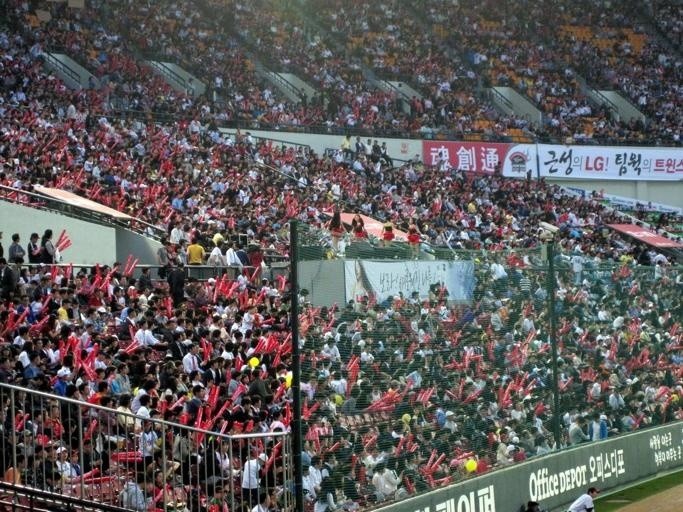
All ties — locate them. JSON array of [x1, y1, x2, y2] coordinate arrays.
[[192, 356, 196, 370]]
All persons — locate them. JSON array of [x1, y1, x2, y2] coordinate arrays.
[[0, 0, 683, 512]]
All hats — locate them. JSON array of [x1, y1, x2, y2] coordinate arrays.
[[445, 411, 455, 418], [150, 409, 176, 420], [167, 361, 177, 370], [215, 486, 227, 493], [56, 446, 67, 453], [22, 429, 32, 435], [208, 278, 216, 283], [32, 233, 40, 239], [57, 369, 69, 377], [259, 454, 268, 460]]
